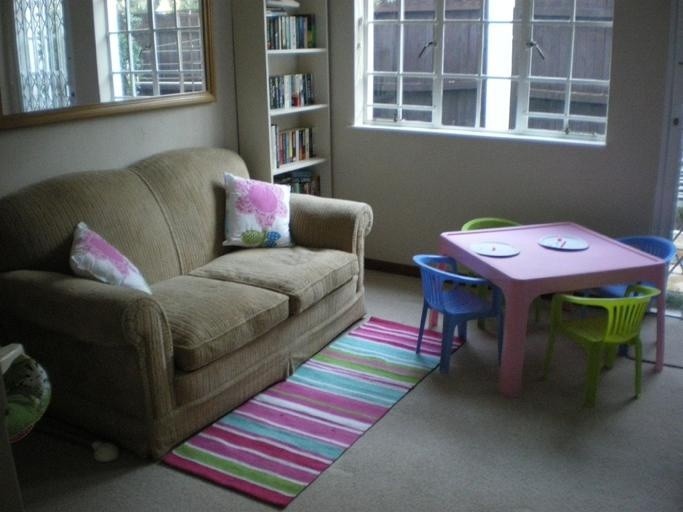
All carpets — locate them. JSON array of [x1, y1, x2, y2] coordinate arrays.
[[158, 315, 467, 510]]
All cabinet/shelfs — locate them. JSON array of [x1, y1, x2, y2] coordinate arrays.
[[232, 0, 333, 198]]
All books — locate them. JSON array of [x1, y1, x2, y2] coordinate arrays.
[[266, 9, 320, 196]]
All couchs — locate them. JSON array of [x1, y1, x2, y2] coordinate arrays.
[[0, 148, 375, 464]]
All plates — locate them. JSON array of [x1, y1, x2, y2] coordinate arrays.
[[537, 233, 589, 251], [471, 240, 520, 257]]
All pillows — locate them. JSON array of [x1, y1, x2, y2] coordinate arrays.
[[69, 220, 153, 297], [221, 170, 296, 250]]
